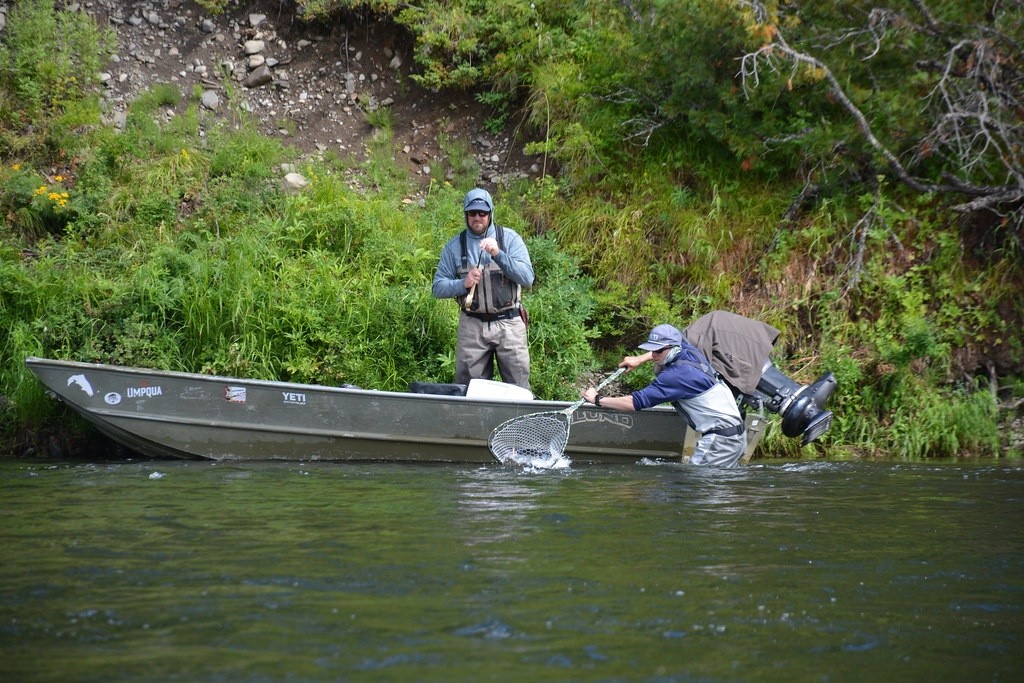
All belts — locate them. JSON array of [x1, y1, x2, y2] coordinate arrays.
[[463, 308, 521, 322], [700, 422, 746, 437]]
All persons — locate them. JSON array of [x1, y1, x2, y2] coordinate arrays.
[[432, 188, 535, 398], [581, 324, 748, 468]]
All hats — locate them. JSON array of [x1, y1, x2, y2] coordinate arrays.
[[638, 324, 682, 351], [464, 199, 491, 212]]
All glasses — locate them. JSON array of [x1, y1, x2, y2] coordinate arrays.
[[468, 210, 488, 217], [652, 347, 673, 353]]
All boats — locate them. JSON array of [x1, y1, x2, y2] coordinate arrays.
[[24, 355, 839, 467]]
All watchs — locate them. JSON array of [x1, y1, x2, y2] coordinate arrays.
[[595, 394, 605, 407]]
